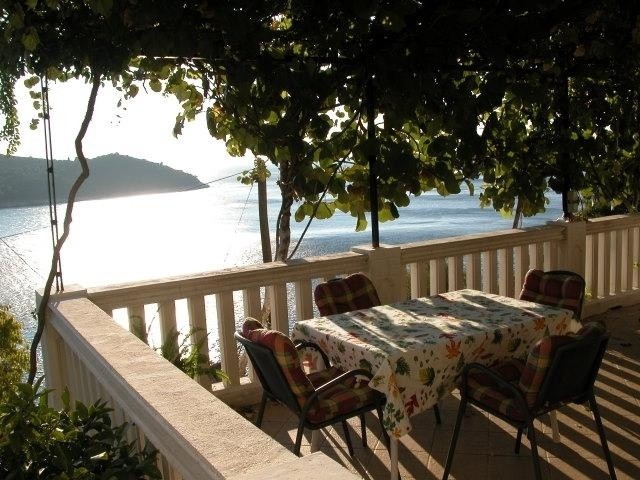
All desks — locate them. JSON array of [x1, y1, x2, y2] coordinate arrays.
[[292, 288, 574, 480]]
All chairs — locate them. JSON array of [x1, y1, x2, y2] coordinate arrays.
[[519, 269, 586, 321], [234, 318, 402, 479], [314, 273, 441, 447], [442, 320, 617, 479]]
